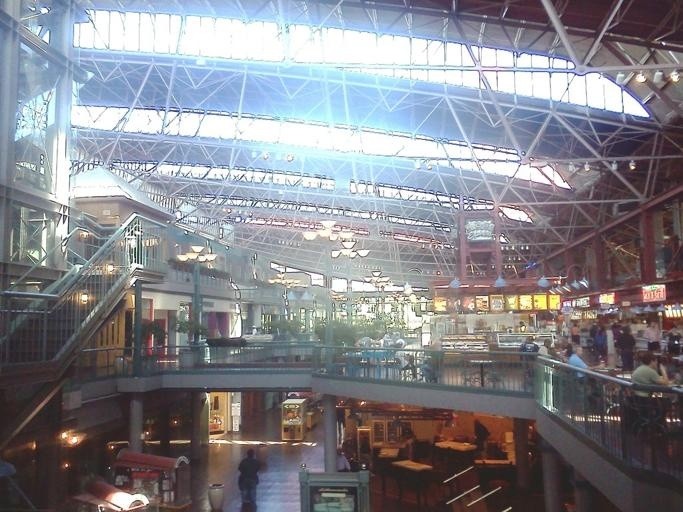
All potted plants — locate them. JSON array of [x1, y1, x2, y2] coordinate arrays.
[[172, 302, 207, 369]]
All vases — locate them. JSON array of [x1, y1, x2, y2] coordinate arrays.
[[207, 482, 224, 511]]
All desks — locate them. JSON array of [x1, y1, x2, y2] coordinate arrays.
[[377, 440, 478, 512]]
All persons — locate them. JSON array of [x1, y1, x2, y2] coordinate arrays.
[[518, 310, 683, 444], [336, 448, 351, 473], [338, 404, 348, 443], [239, 448, 263, 511], [395, 419, 416, 460], [474, 419, 489, 451], [356, 328, 441, 381]]
